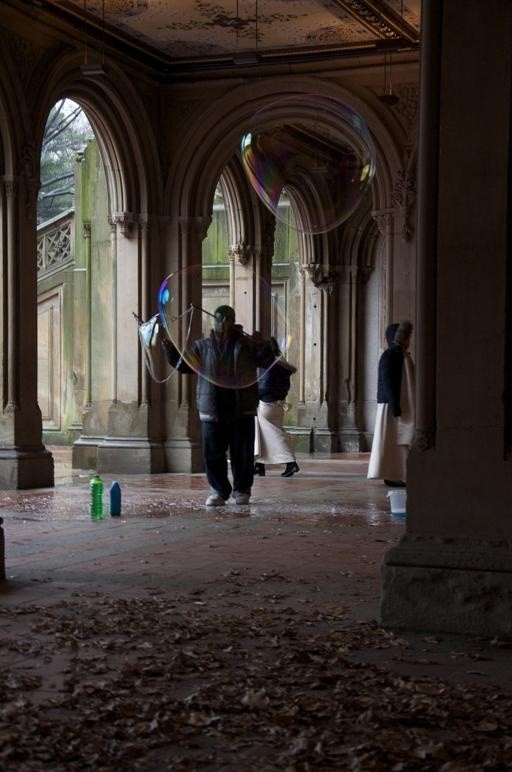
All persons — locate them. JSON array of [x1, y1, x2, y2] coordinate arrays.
[[161, 305, 299, 506], [367, 320, 416, 487]]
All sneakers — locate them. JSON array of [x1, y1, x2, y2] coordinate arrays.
[[232, 491, 251, 505], [205, 492, 225, 506]]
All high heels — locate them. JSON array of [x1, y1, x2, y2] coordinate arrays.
[[281, 462, 299, 477], [254, 463, 265, 476]]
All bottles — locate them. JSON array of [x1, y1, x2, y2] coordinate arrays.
[[88, 475, 103, 521]]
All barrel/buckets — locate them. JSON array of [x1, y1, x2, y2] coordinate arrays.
[[387, 489, 408, 513]]
